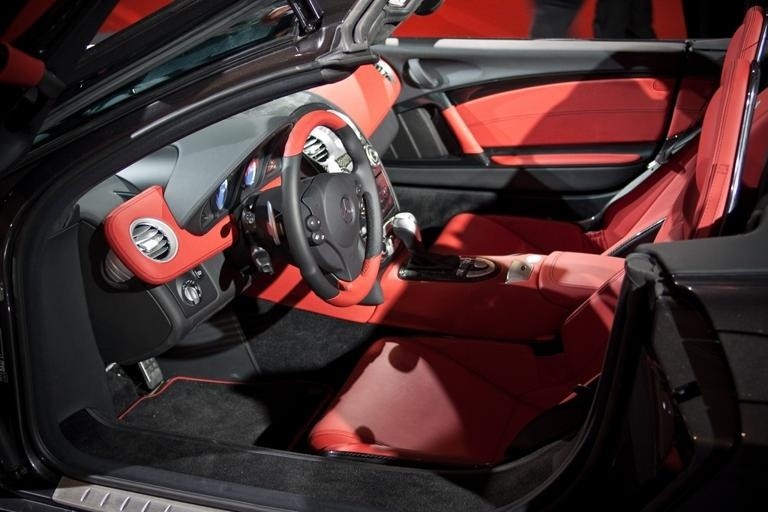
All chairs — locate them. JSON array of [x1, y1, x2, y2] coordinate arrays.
[[307, 6, 768, 470], [425, 87, 768, 255]]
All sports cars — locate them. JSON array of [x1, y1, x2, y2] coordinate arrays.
[[0, 1, 767, 512]]
